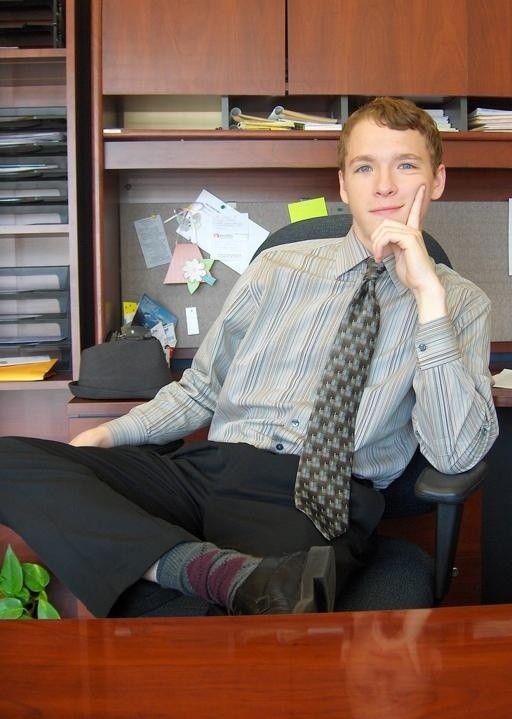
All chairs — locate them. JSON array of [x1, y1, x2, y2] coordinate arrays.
[[225, 213, 491, 612]]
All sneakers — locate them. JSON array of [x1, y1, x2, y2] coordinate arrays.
[[228, 546, 336, 614]]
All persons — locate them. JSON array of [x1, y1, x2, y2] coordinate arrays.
[[1, 92, 503, 620]]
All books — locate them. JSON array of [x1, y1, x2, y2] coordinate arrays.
[[227, 105, 342, 132], [419, 106, 511, 134]]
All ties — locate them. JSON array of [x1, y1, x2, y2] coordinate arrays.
[[292, 259, 388, 546]]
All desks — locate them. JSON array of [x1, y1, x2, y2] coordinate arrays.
[[2, 606, 508, 719]]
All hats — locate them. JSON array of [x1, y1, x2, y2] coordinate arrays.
[[67, 336, 174, 401]]
